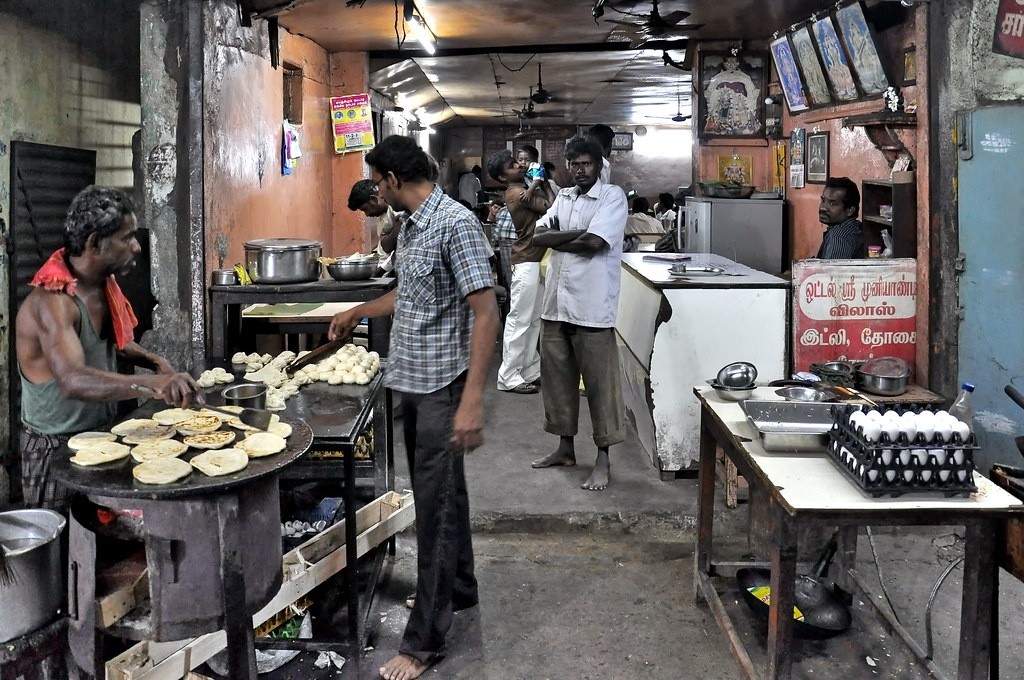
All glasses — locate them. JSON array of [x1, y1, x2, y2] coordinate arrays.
[[370, 170, 392, 195]]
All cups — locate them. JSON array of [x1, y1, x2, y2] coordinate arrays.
[[868, 246, 881, 257]]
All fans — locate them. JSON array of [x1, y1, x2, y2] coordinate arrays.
[[494, 62, 568, 139], [644, 86, 692, 122], [602, 0, 707, 49]]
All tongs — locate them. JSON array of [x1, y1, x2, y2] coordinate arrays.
[[284, 335, 351, 375]]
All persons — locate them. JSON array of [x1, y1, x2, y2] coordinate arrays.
[[816, 177, 863, 259], [589, 124, 615, 184], [487, 144, 556, 393], [458, 165, 481, 213], [654, 193, 676, 231], [531, 136, 628, 490], [16, 186, 201, 512], [481, 200, 504, 224], [347, 153, 439, 269], [625, 197, 666, 242], [493, 206, 518, 241], [329, 135, 500, 680]]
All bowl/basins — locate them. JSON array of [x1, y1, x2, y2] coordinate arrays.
[[221, 383, 269, 410], [774, 356, 911, 402], [705, 361, 758, 401], [326, 261, 378, 281], [698, 183, 756, 199], [213, 269, 241, 285]]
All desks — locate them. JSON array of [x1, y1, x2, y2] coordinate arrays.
[[244, 302, 365, 354], [134, 360, 397, 667], [208, 275, 394, 488]]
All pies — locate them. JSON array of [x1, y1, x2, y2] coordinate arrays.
[[66, 404, 292, 484]]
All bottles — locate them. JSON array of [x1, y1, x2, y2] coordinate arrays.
[[947, 382, 975, 433]]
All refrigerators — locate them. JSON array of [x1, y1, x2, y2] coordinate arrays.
[[677, 197, 783, 275]]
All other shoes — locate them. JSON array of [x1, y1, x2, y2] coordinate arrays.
[[505, 383, 537, 393], [531, 377, 541, 386]]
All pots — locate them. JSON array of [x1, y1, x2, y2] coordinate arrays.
[[1003, 383, 1024, 458], [242, 237, 324, 285], [734, 540, 853, 637]]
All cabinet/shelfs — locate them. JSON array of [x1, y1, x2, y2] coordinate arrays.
[[860, 177, 920, 260], [690, 384, 1023, 680]]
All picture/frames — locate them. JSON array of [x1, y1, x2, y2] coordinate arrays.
[[697, 48, 772, 141], [717, 155, 752, 184], [806, 130, 832, 183]]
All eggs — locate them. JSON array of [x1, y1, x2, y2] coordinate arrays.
[[834, 410, 970, 481]]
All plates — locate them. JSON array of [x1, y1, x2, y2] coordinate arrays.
[[667, 266, 724, 276]]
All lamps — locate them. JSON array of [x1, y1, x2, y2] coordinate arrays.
[[403, 0, 438, 54]]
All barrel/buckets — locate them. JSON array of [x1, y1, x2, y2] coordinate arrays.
[[0, 508, 66, 645]]
